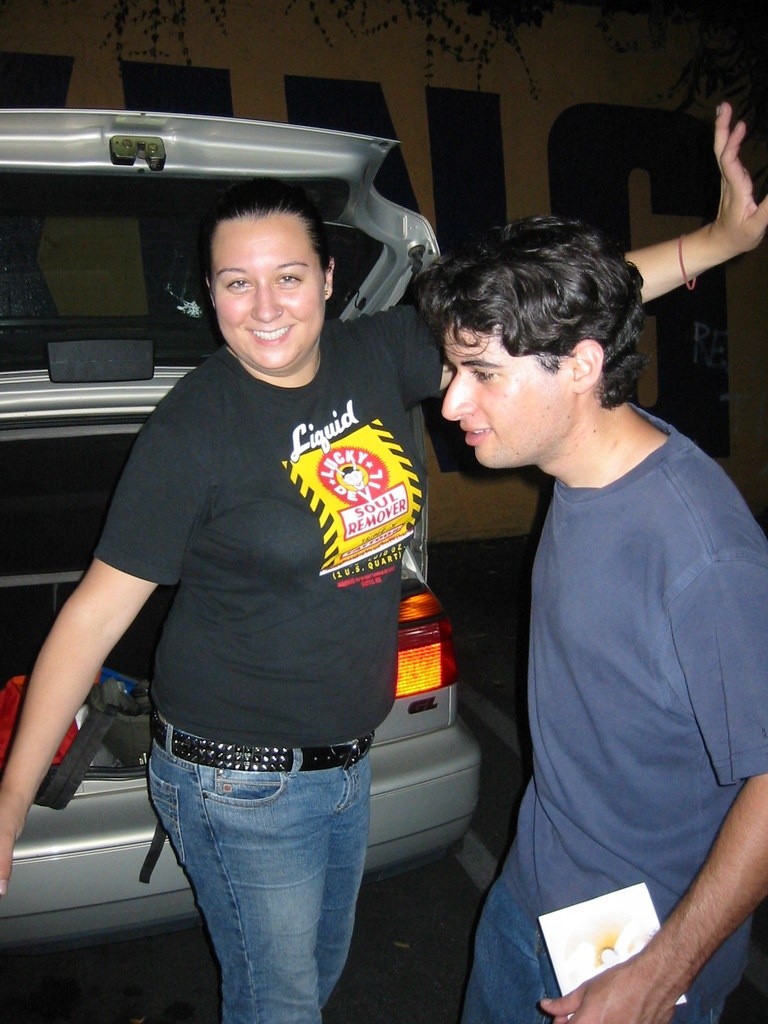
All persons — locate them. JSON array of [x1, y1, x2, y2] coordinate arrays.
[[414, 215, 768, 1024], [0, 100, 768, 1023]]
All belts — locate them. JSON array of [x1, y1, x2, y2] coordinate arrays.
[[151, 708, 375, 772]]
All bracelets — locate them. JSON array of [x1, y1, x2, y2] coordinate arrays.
[[678, 233, 696, 287]]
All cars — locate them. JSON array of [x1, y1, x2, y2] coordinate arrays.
[[0, 107, 489, 951]]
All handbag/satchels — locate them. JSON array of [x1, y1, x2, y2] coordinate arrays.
[[34, 677, 151, 809]]
